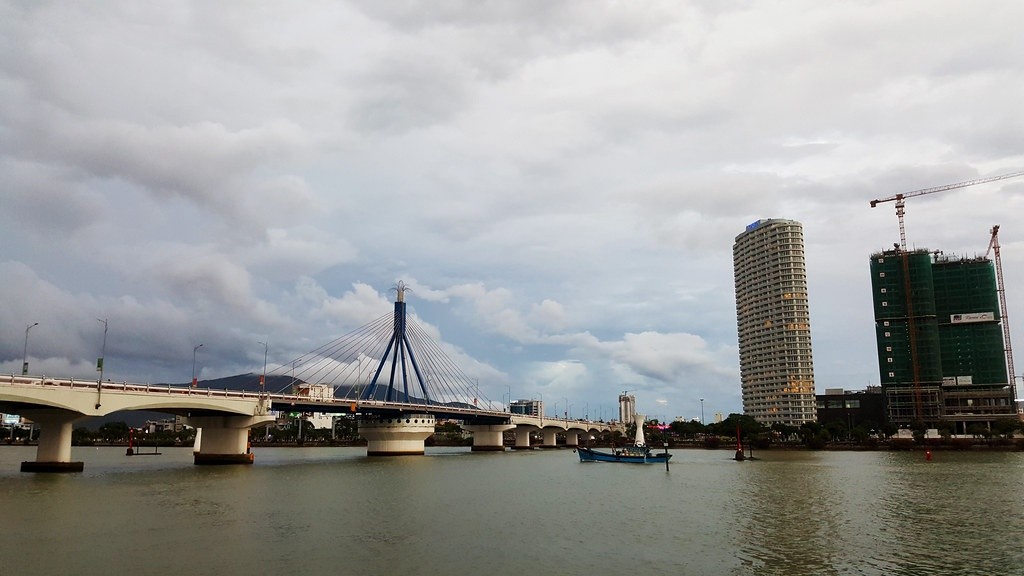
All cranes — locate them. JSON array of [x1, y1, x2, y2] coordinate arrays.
[[984, 226, 1019, 419], [869, 163, 1024, 418]]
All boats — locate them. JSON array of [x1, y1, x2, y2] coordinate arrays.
[[126, 428, 162, 456], [736, 421, 756, 460], [575, 445, 672, 463]]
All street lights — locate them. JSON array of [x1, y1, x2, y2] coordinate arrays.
[[354, 357, 361, 399], [538, 393, 543, 400], [22, 322, 39, 376], [700, 398, 704, 424], [502, 384, 512, 413], [580, 402, 590, 420], [467, 378, 478, 410], [96, 316, 109, 380], [193, 343, 204, 388], [554, 398, 575, 420], [293, 359, 302, 390], [258, 341, 269, 392], [594, 405, 615, 423]]
